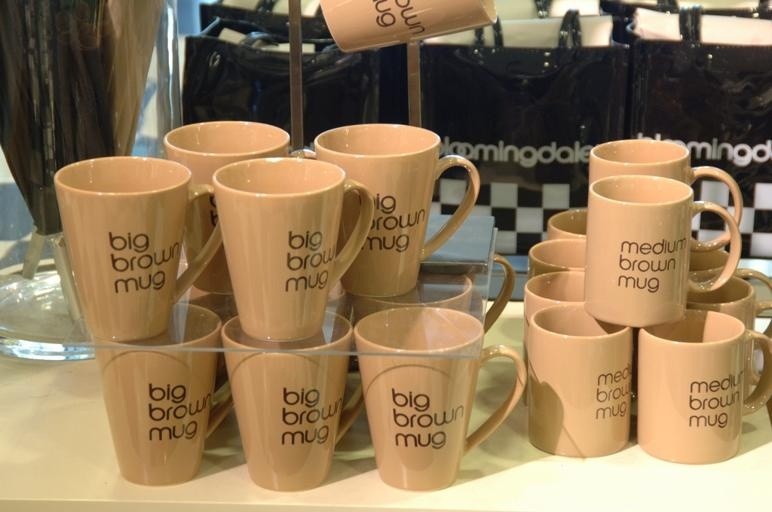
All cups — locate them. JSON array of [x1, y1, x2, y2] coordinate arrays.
[[92, 303, 233, 487], [315, 121, 483, 299], [163, 120, 318, 296], [546, 210, 586, 239], [688, 248, 731, 274], [318, 0, 498, 55], [686, 270, 772, 338], [582, 174, 742, 327], [524, 306, 634, 457], [212, 156, 374, 341], [523, 270, 586, 407], [53, 156, 222, 343], [222, 311, 364, 493], [354, 253, 515, 334], [528, 241, 589, 278], [635, 308, 772, 466], [587, 139, 743, 252], [326, 287, 351, 316], [354, 307, 528, 492]]
